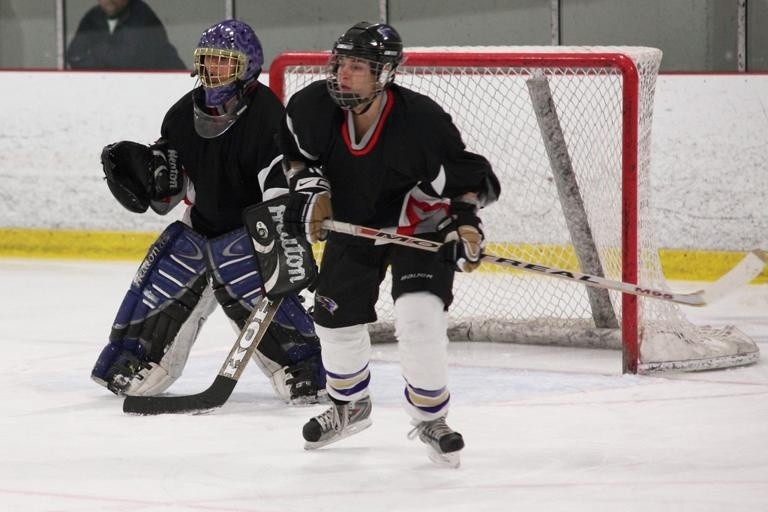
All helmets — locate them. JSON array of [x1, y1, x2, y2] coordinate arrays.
[[188, 19, 265, 110], [332, 19, 404, 65]]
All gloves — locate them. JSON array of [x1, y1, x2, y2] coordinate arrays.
[[282, 164, 336, 250], [434, 201, 488, 275]]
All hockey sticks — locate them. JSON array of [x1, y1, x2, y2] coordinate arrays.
[[321, 219, 768, 307], [123, 297, 283, 414]]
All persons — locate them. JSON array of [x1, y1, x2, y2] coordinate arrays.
[[274, 22, 481, 469], [67, 0, 189, 70], [91, 18, 330, 409]]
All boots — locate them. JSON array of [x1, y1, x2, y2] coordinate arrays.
[[300, 391, 373, 443], [409, 411, 468, 456]]
